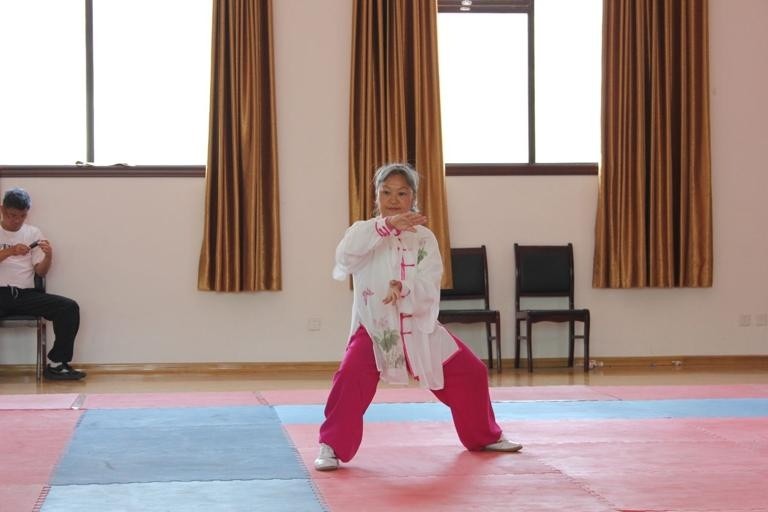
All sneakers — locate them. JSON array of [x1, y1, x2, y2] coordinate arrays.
[[43, 362, 87, 381], [314, 442, 340, 472], [485, 433, 523, 453]]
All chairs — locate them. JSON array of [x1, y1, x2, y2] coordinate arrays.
[[513, 243, 592, 375], [421, 246, 503, 375], [0, 270, 49, 380]]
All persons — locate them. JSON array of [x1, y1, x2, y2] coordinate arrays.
[[313, 164, 523, 471], [0, 186, 87, 381]]
